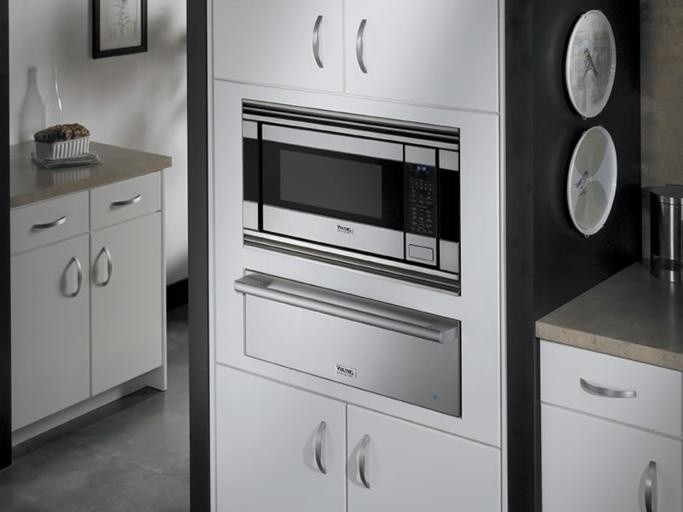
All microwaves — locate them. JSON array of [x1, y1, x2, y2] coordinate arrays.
[[242, 99, 461, 297]]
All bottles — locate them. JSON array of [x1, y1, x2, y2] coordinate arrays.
[[43, 63, 63, 129]]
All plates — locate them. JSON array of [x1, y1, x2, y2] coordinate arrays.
[[565, 6, 620, 245]]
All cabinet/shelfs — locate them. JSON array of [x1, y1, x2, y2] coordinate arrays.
[[207, 360, 507, 512], [207, 0, 506, 115], [10, 170, 167, 459], [539, 338, 683, 512]]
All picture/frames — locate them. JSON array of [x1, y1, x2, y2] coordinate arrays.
[[92, 0, 148, 59]]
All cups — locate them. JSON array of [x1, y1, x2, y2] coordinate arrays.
[[646, 182, 683, 284]]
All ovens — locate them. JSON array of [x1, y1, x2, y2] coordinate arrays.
[[231, 273, 463, 421]]
